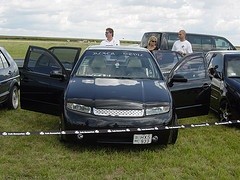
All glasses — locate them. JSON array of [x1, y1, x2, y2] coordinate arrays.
[[178, 34, 181, 35], [152, 41, 156, 43], [106, 32, 109, 34]]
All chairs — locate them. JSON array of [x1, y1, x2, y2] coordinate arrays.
[[91, 55, 107, 69], [127, 58, 146, 72]]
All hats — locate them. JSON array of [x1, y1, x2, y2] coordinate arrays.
[[215, 65, 218, 68]]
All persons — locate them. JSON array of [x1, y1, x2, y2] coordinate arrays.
[[170, 29, 194, 70], [139, 35, 162, 62], [95, 27, 121, 61]]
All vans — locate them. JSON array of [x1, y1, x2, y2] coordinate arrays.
[[140, 32, 237, 58]]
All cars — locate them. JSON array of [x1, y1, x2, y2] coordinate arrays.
[[198, 50, 240, 123], [20, 45, 211, 149], [0, 46, 20, 111]]
